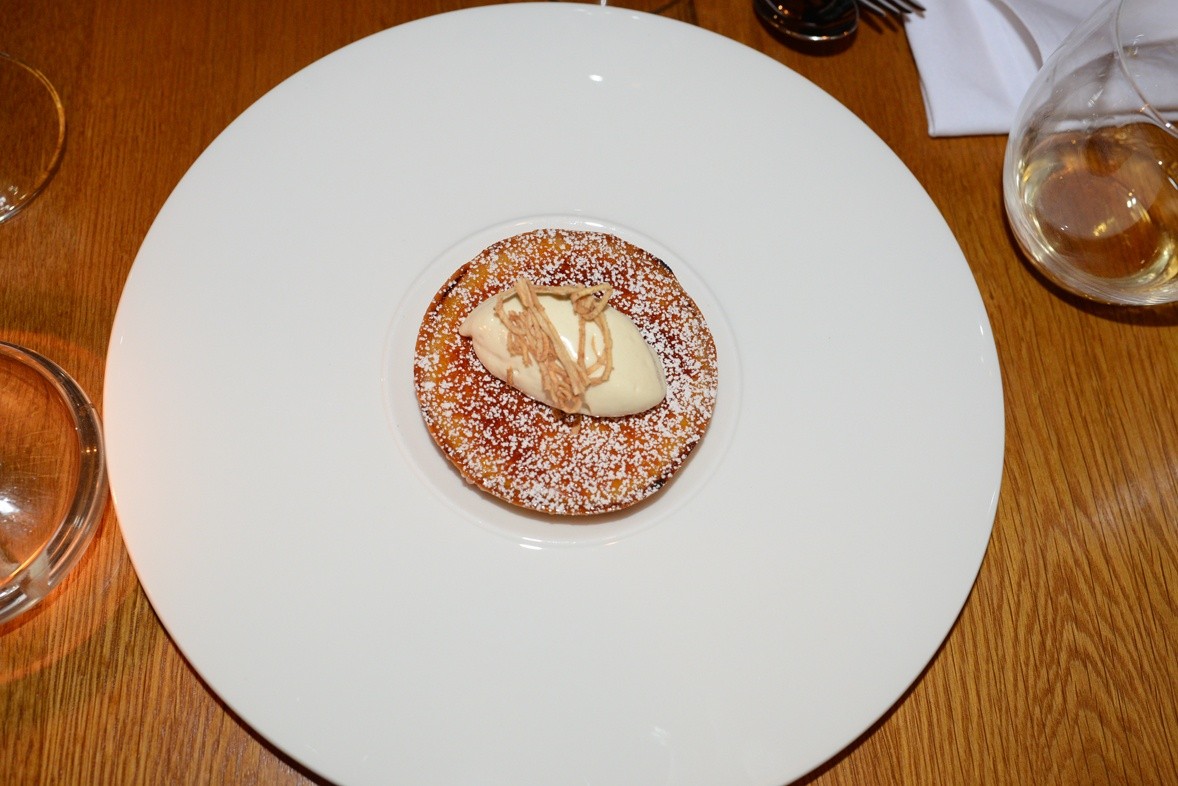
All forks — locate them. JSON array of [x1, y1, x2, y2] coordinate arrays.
[[860, 0, 927, 18]]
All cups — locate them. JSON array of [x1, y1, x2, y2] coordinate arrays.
[[998, 0, 1178, 312]]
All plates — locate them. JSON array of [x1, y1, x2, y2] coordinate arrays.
[[102, 0, 1011, 784]]
